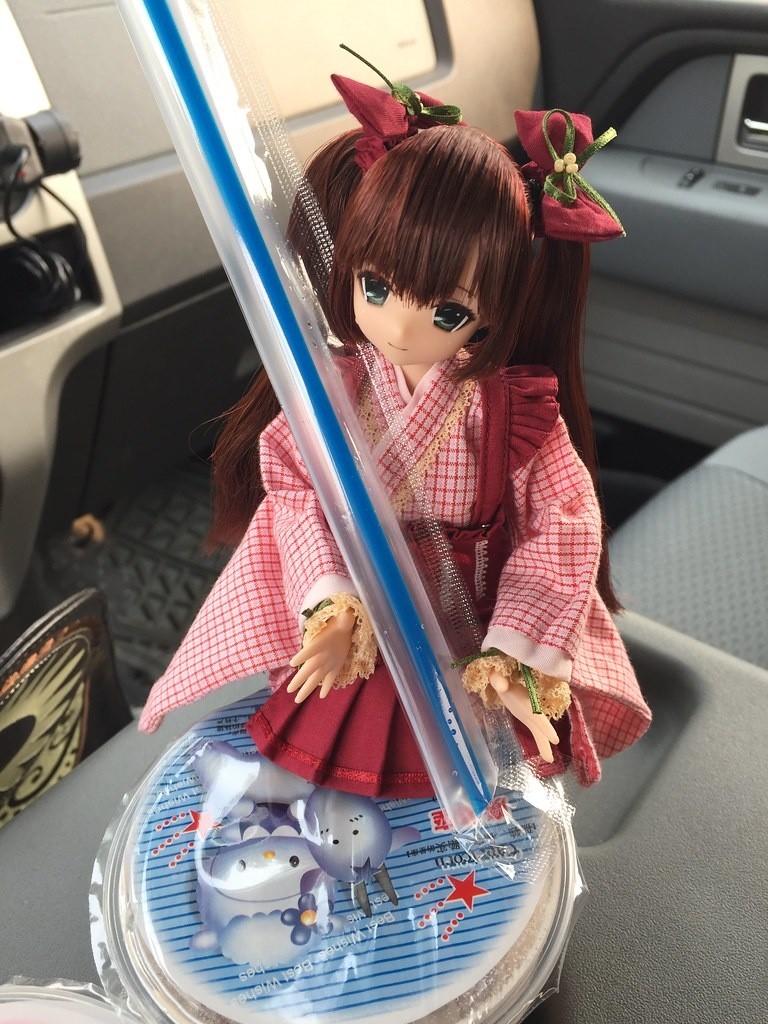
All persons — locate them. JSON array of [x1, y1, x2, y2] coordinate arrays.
[[204, 41, 656, 802]]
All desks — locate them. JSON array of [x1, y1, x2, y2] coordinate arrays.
[[0, 598, 768, 1023]]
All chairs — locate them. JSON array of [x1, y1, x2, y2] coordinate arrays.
[[1, 265, 267, 709]]
